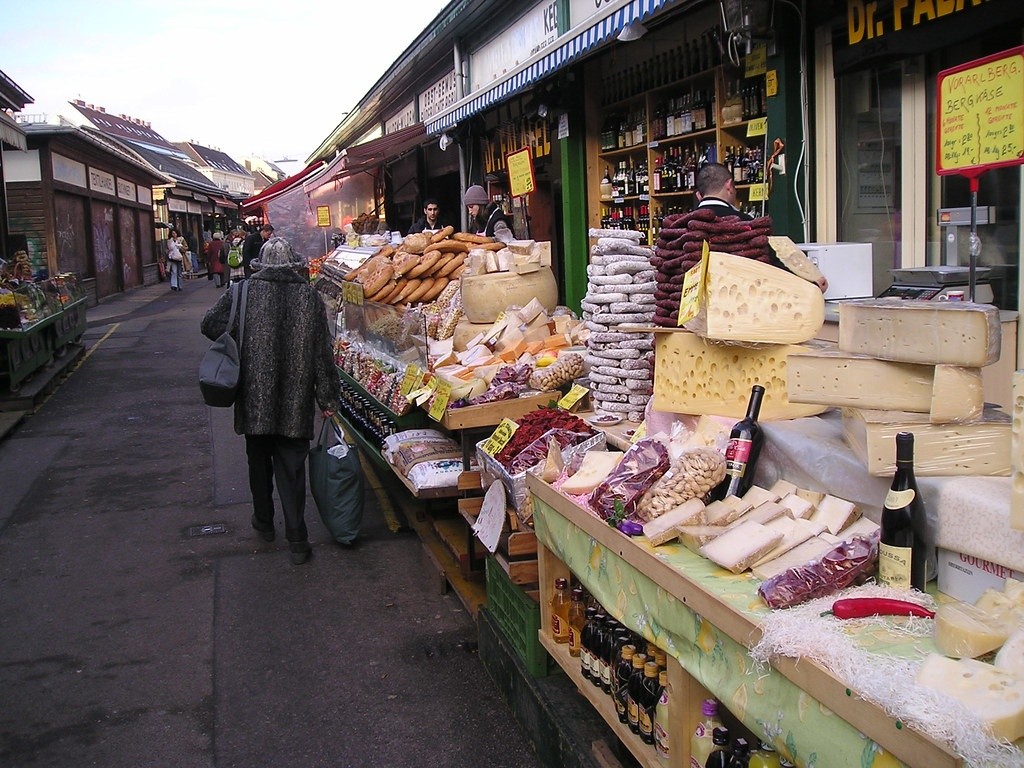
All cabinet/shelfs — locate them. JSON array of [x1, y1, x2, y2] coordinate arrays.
[[321, 302, 1024, 768], [596, 58, 770, 249]]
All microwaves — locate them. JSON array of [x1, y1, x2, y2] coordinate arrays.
[[795, 242, 872, 299]]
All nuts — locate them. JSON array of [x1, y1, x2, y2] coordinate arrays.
[[529, 352, 586, 391], [634, 449, 727, 517]]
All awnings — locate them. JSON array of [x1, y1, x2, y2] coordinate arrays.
[[240, 161, 326, 212], [424, 0, 674, 136], [208, 196, 238, 209], [303, 119, 429, 194]]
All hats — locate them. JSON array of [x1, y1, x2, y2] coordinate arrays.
[[250, 236, 306, 270], [464, 185, 489, 206]]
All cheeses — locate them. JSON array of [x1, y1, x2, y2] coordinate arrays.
[[644, 478, 880, 580], [920, 575, 1023, 744], [652, 236, 1024, 533], [433, 297, 549, 402], [542, 314, 591, 349], [469, 239, 551, 274]]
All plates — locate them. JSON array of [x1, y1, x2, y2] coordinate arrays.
[[588, 415, 623, 426], [620, 429, 638, 439]]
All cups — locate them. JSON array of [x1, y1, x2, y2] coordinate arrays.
[[939, 291, 964, 302], [600, 183, 612, 199], [721, 105, 742, 124]]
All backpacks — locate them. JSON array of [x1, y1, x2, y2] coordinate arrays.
[[227, 239, 243, 267]]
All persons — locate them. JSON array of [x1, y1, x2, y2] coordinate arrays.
[[406, 199, 444, 235], [243, 224, 273, 280], [185, 231, 199, 273], [463, 185, 516, 240], [695, 163, 754, 222], [204, 220, 257, 290], [199, 237, 342, 564], [166, 230, 188, 291]]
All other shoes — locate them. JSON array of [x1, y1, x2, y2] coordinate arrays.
[[171, 287, 177, 291]]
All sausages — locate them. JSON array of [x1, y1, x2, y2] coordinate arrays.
[[587, 228, 660, 423], [648, 208, 778, 330]]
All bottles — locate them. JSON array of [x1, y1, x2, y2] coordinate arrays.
[[601, 106, 647, 152], [604, 155, 648, 198], [615, 636, 670, 759], [552, 577, 572, 644], [726, 77, 767, 118], [601, 23, 721, 108], [878, 432, 927, 593], [652, 81, 716, 140], [652, 201, 693, 245], [581, 607, 596, 679], [609, 628, 625, 699], [654, 138, 716, 193], [724, 145, 767, 219], [711, 385, 765, 501], [569, 589, 587, 657], [338, 379, 397, 438], [690, 698, 796, 768], [601, 205, 649, 245], [590, 614, 607, 687], [600, 620, 618, 694]]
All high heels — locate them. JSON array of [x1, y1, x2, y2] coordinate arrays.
[[289, 540, 311, 564], [251, 514, 275, 541]]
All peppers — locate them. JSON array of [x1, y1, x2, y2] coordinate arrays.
[[758, 541, 874, 611], [458, 364, 595, 474], [819, 598, 935, 620]]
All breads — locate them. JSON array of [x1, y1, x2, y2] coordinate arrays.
[[342, 226, 506, 323]]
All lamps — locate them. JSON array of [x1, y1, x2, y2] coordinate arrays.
[[617, 19, 647, 40], [439, 127, 462, 151]]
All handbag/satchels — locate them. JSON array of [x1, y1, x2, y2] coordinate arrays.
[[308, 415, 364, 545], [168, 246, 182, 262], [198, 280, 248, 407]]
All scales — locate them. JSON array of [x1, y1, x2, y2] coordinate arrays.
[[875, 264, 995, 303]]
